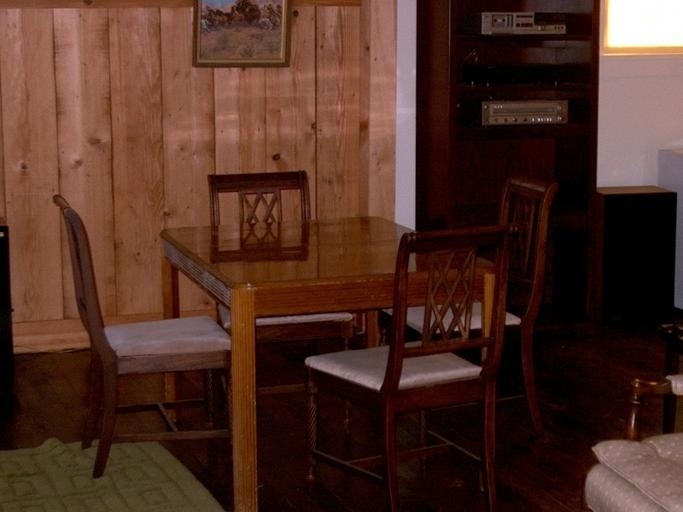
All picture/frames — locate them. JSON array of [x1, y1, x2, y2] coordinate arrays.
[[192, 2, 292, 69]]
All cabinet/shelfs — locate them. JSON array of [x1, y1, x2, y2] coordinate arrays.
[[417, 1, 599, 340]]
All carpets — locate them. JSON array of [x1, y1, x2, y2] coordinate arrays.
[[1, 440, 226, 512]]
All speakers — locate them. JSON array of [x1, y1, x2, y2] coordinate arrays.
[[591, 184, 677, 333]]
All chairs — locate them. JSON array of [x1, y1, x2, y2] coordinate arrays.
[[580, 372, 682, 512], [206, 171, 355, 450], [301, 222, 520, 512], [53, 194, 232, 478], [376, 173, 558, 436], [210, 218, 310, 265]]
